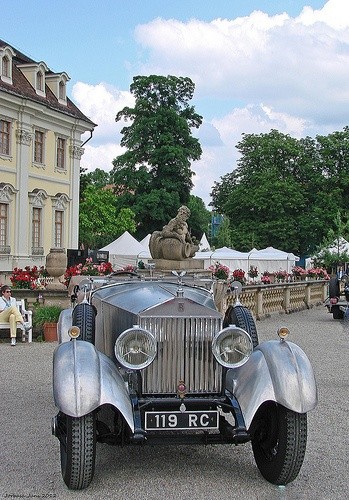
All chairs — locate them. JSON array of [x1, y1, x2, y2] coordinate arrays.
[[0, 299, 33, 343]]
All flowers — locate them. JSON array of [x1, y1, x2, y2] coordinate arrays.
[[248, 266, 258, 278], [233, 269, 246, 286], [261, 276, 272, 284], [208, 264, 229, 280], [292, 266, 330, 280], [276, 270, 288, 280]]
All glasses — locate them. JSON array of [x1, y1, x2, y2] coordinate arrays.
[[5, 291, 12, 293]]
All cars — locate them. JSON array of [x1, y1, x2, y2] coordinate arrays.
[[51, 268, 318, 490], [324, 266, 349, 320]]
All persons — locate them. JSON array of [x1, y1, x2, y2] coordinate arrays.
[[341, 275, 349, 302], [162, 205, 191, 244], [0, 285, 33, 346]]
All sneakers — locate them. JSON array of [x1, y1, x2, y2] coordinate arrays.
[[11, 339, 16, 346], [24, 326, 33, 331]]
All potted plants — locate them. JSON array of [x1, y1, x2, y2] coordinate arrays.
[[33, 306, 65, 342]]
